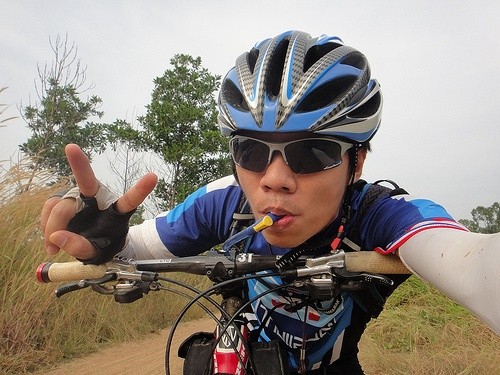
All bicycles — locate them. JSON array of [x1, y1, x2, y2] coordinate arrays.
[[35, 239, 412, 374]]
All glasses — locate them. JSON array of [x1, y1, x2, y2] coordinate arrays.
[[226, 135, 361, 174]]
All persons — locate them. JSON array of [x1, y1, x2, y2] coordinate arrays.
[[41, 31, 500, 375]]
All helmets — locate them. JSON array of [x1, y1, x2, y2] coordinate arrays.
[[217, 30, 384, 144]]
